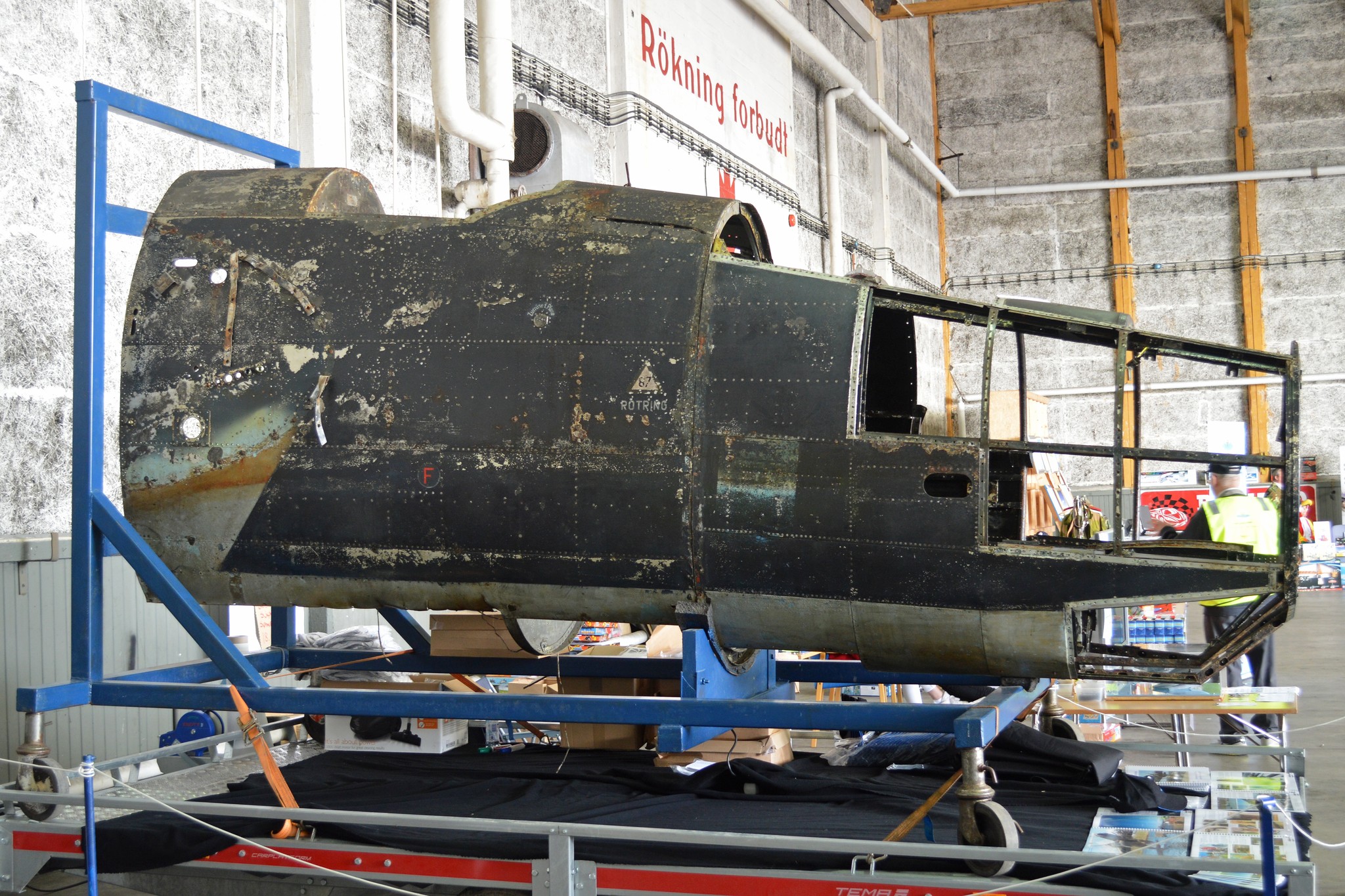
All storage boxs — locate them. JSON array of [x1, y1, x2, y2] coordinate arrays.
[[578, 628, 605, 635], [655, 727, 795, 769], [1057, 680, 1073, 696], [508, 678, 558, 694], [582, 621, 617, 628], [1074, 684, 1105, 701], [1080, 723, 1121, 742], [430, 611, 553, 659], [557, 644, 647, 752], [321, 675, 493, 753], [574, 634, 609, 642], [569, 643, 620, 651]]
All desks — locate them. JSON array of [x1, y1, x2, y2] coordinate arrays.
[[1059, 684, 1299, 773]]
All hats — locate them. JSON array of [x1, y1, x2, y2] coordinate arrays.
[[1203, 463, 1241, 474]]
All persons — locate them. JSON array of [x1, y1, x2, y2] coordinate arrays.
[[1151, 462, 1289, 755]]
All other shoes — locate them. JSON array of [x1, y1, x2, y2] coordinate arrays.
[[1209, 737, 1248, 755], [1261, 736, 1279, 746]]
